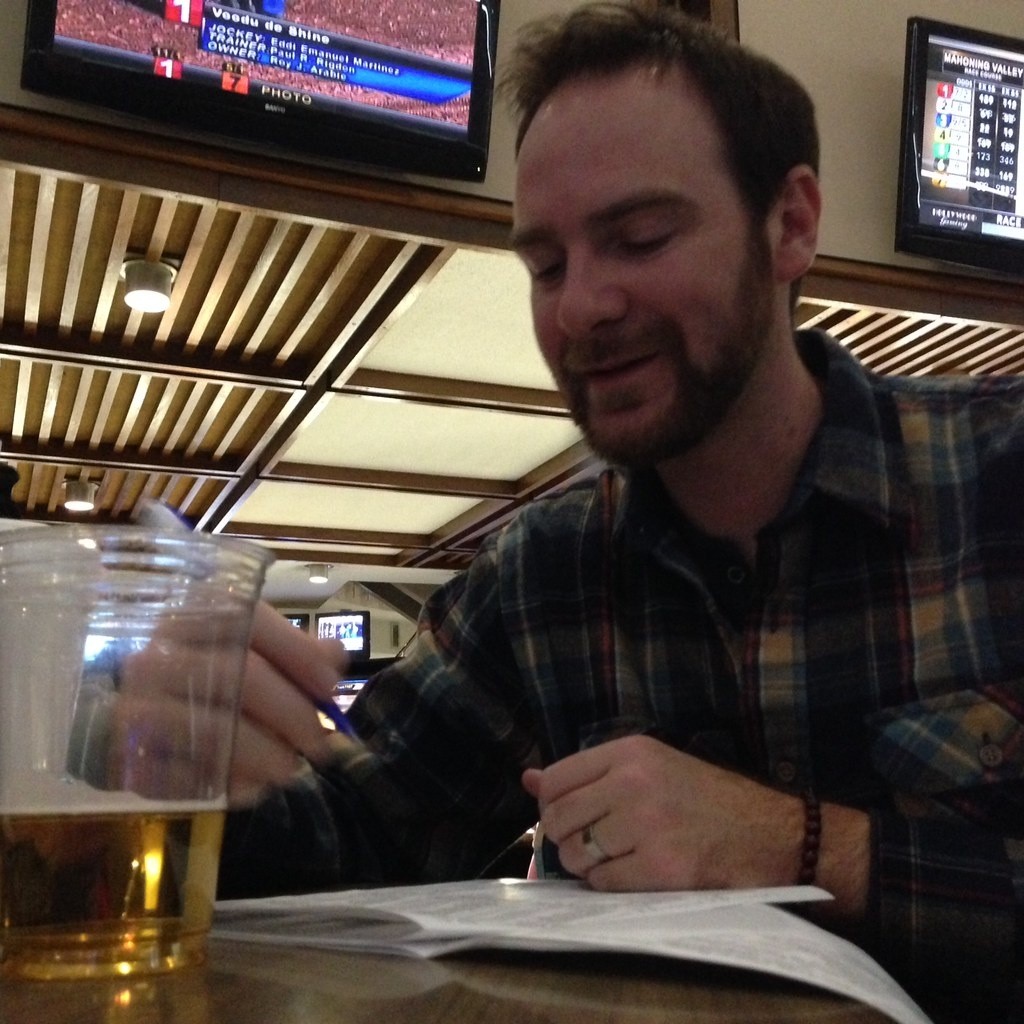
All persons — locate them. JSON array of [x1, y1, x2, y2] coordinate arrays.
[[108, 0, 1024, 1024]]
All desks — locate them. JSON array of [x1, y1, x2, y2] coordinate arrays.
[[0, 906, 893, 1024]]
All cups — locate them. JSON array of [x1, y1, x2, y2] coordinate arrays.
[[1, 526, 277, 978]]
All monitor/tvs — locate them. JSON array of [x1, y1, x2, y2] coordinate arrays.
[[282, 613, 310, 633], [315, 610, 371, 659], [21, 0, 501, 182], [894, 15, 1024, 274]]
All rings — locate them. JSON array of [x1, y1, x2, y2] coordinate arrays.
[[579, 824, 610, 862]]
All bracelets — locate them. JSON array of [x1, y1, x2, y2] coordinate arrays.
[[797, 789, 825, 887]]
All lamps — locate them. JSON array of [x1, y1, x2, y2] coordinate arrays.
[[61, 481, 100, 512], [119, 258, 179, 313], [303, 564, 332, 582]]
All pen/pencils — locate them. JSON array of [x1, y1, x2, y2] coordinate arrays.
[[124, 495, 373, 764]]
